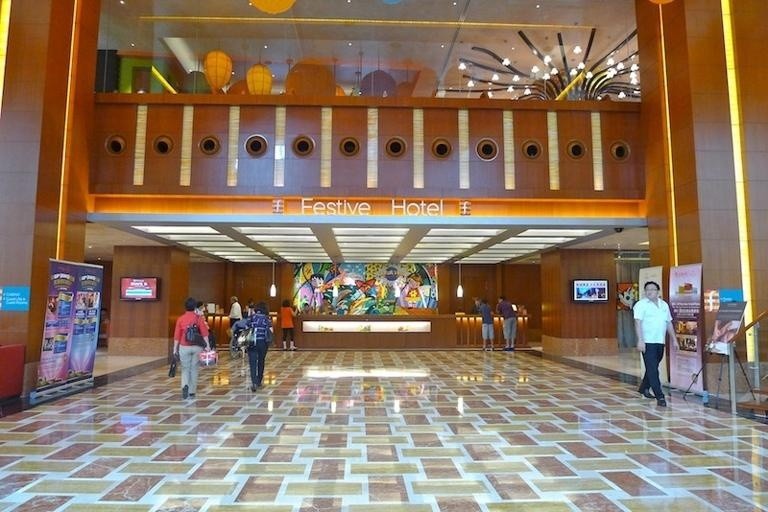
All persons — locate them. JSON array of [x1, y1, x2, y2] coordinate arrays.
[[277, 300, 299, 350], [633, 282, 679, 407], [496, 295, 517, 350], [98, 308, 110, 348], [195, 301, 210, 331], [302, 303, 313, 314], [228, 296, 256, 351], [711, 319, 742, 343], [244, 302, 274, 392], [471, 298, 482, 315], [319, 296, 335, 315], [479, 297, 495, 351], [172, 298, 210, 399]]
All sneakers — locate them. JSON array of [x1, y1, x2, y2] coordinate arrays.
[[181, 385, 197, 400], [503, 346, 515, 351], [482, 346, 495, 352], [249, 379, 263, 392]]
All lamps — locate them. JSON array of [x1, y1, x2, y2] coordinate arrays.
[[174, 33, 442, 101], [455, 263, 464, 297], [270, 261, 277, 297]]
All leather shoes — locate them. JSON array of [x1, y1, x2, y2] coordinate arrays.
[[640, 388, 656, 399], [657, 397, 667, 406]]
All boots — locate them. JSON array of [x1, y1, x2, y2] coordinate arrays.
[[282, 340, 298, 351]]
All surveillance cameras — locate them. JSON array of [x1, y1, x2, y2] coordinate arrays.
[[615, 228, 624, 232]]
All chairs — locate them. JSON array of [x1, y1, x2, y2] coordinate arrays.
[[0, 342, 28, 398]]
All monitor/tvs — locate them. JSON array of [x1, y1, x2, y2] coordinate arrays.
[[573, 279, 609, 304], [119, 277, 160, 302]]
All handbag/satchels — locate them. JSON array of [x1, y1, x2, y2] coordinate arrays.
[[185, 326, 205, 345], [168, 355, 178, 378], [199, 349, 217, 366], [234, 330, 248, 348]]
